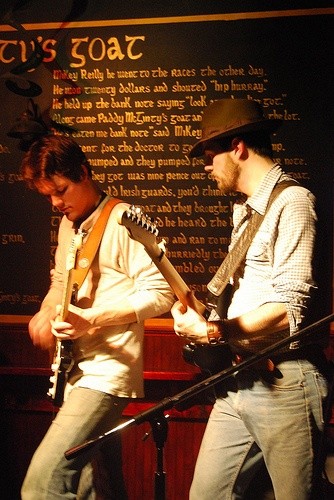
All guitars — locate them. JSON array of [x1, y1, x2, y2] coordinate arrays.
[[46, 229, 88, 407], [117, 203, 282, 375]]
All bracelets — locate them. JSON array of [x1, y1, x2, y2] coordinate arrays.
[[207, 321, 229, 346]]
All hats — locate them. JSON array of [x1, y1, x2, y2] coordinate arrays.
[[187, 99, 305, 158]]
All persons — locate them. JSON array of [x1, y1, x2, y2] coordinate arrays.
[[170, 98, 330, 500], [21, 136, 174, 500]]
